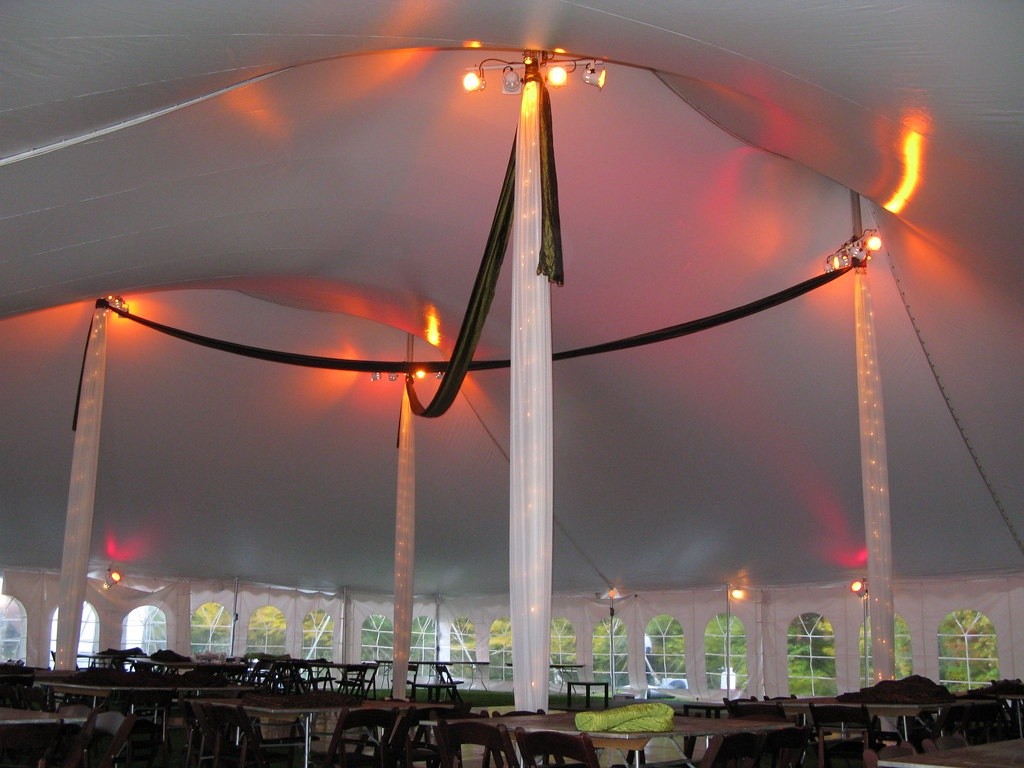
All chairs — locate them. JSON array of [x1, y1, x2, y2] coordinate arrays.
[[0, 650, 1024, 768]]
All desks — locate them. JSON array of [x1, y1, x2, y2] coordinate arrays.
[[258, 659, 371, 668], [374, 658, 490, 692], [77, 653, 147, 660], [126, 657, 249, 671], [414, 710, 797, 742], [37, 678, 255, 702], [504, 661, 585, 695], [190, 694, 455, 717], [737, 690, 995, 718]]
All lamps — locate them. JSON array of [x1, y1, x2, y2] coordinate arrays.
[[500, 69, 521, 94], [848, 579, 868, 599], [850, 247, 867, 262], [834, 254, 851, 269], [105, 570, 121, 587], [584, 63, 605, 89]]
